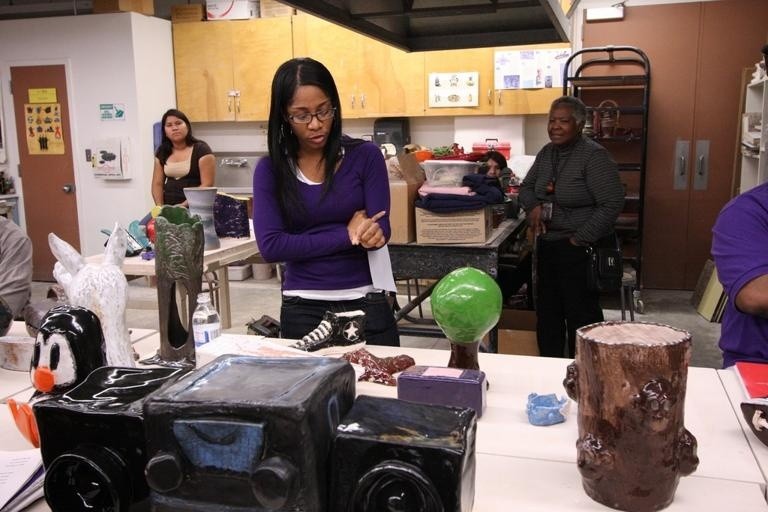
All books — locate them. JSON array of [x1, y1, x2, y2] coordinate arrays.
[[734, 361, 768, 405]]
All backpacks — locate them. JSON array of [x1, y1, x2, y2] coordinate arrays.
[[582, 245, 624, 293]]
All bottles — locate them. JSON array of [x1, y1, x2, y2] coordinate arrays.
[[536, 69, 542, 86], [545, 66, 552, 88], [363, 288, 402, 347], [192, 292, 223, 348]]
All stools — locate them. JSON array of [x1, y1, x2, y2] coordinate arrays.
[[618, 272, 637, 323]]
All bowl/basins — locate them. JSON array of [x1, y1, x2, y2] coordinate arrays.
[[421, 160, 481, 186]]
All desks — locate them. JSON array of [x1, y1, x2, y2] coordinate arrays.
[[377, 204, 539, 352], [0, 311, 766, 511], [108, 214, 282, 324]]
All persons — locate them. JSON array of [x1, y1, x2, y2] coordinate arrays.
[[711, 183, 768, 368], [520, 95, 626, 359], [139, 108, 216, 227], [0, 215, 34, 337], [480, 150, 507, 178], [253, 56, 400, 346]]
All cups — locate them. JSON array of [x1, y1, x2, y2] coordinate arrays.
[[0, 337, 38, 372], [503, 73, 520, 89]]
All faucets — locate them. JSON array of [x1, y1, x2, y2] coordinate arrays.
[[218, 158, 248, 168]]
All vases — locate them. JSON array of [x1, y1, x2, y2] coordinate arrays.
[[182, 184, 221, 253]]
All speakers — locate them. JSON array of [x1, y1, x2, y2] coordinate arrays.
[[325, 395, 477, 512], [30, 364, 193, 512]]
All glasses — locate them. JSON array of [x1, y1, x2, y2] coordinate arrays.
[[285, 107, 336, 124]]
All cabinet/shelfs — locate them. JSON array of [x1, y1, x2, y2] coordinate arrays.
[[174, 15, 290, 126], [292, 15, 405, 125], [425, 2, 575, 117], [732, 76, 768, 203], [584, 0, 767, 289]]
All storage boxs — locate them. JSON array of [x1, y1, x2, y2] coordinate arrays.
[[472, 136, 512, 161], [208, 261, 252, 282], [374, 153, 416, 244], [478, 310, 541, 357], [89, 2, 307, 24], [417, 200, 493, 246], [420, 158, 484, 187]]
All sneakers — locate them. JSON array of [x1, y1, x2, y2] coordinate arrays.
[[277, 309, 367, 359]]
